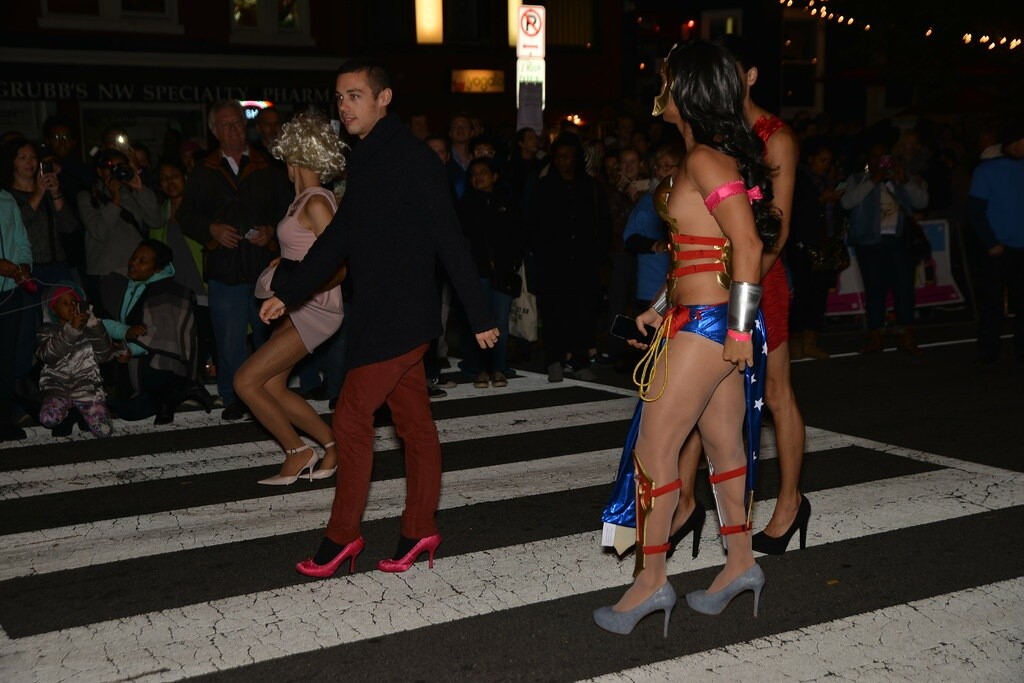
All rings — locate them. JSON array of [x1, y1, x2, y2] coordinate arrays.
[[493, 338, 498, 343]]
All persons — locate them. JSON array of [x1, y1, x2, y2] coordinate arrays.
[[256, 57, 501, 576], [592, 34, 811, 637], [231, 113, 350, 484], [0, 99, 1024, 436]]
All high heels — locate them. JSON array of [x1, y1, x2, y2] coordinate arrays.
[[258, 444, 318, 484], [751, 492, 811, 553], [686, 563, 764, 617], [379, 534, 440, 573], [299, 441, 337, 478], [296, 538, 364, 575], [594, 581, 677, 638], [666, 504, 706, 558]]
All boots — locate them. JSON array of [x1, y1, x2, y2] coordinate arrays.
[[789, 333, 805, 358], [900, 327, 922, 357], [859, 329, 882, 352], [804, 329, 830, 358]]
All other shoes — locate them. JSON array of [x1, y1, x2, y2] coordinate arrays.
[[222, 409, 243, 419], [974, 346, 1000, 362], [3, 426, 28, 439], [52, 411, 71, 437], [77, 412, 92, 431], [154, 413, 174, 424]]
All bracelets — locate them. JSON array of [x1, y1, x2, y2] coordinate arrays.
[[727, 330, 750, 342]]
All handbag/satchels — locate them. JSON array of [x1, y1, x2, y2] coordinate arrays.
[[508, 263, 538, 343]]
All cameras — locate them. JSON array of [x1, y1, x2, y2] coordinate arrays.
[[110, 165, 135, 182]]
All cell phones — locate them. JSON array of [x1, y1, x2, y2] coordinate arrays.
[[610, 314, 657, 345], [880, 155, 895, 173], [836, 181, 850, 192], [245, 229, 260, 240], [40, 161, 53, 176]]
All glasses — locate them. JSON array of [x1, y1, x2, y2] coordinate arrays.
[[654, 164, 680, 171], [47, 135, 73, 143], [474, 150, 496, 156]]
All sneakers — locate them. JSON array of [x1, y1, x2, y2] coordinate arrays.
[[590, 352, 615, 368], [574, 368, 596, 381], [474, 373, 489, 387], [491, 372, 506, 387], [427, 386, 446, 398], [548, 364, 563, 383]]
